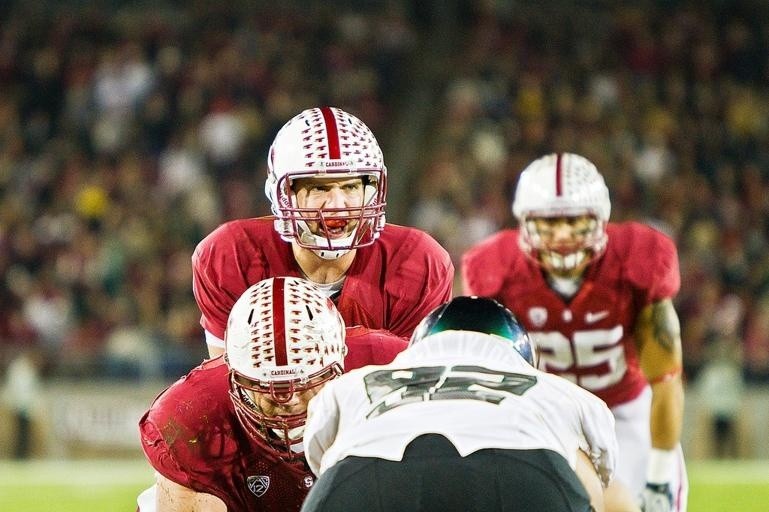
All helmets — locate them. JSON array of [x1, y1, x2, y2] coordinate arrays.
[[223, 274, 349, 467], [264, 106, 389, 262], [406, 295, 536, 368], [511, 151, 612, 273]]
